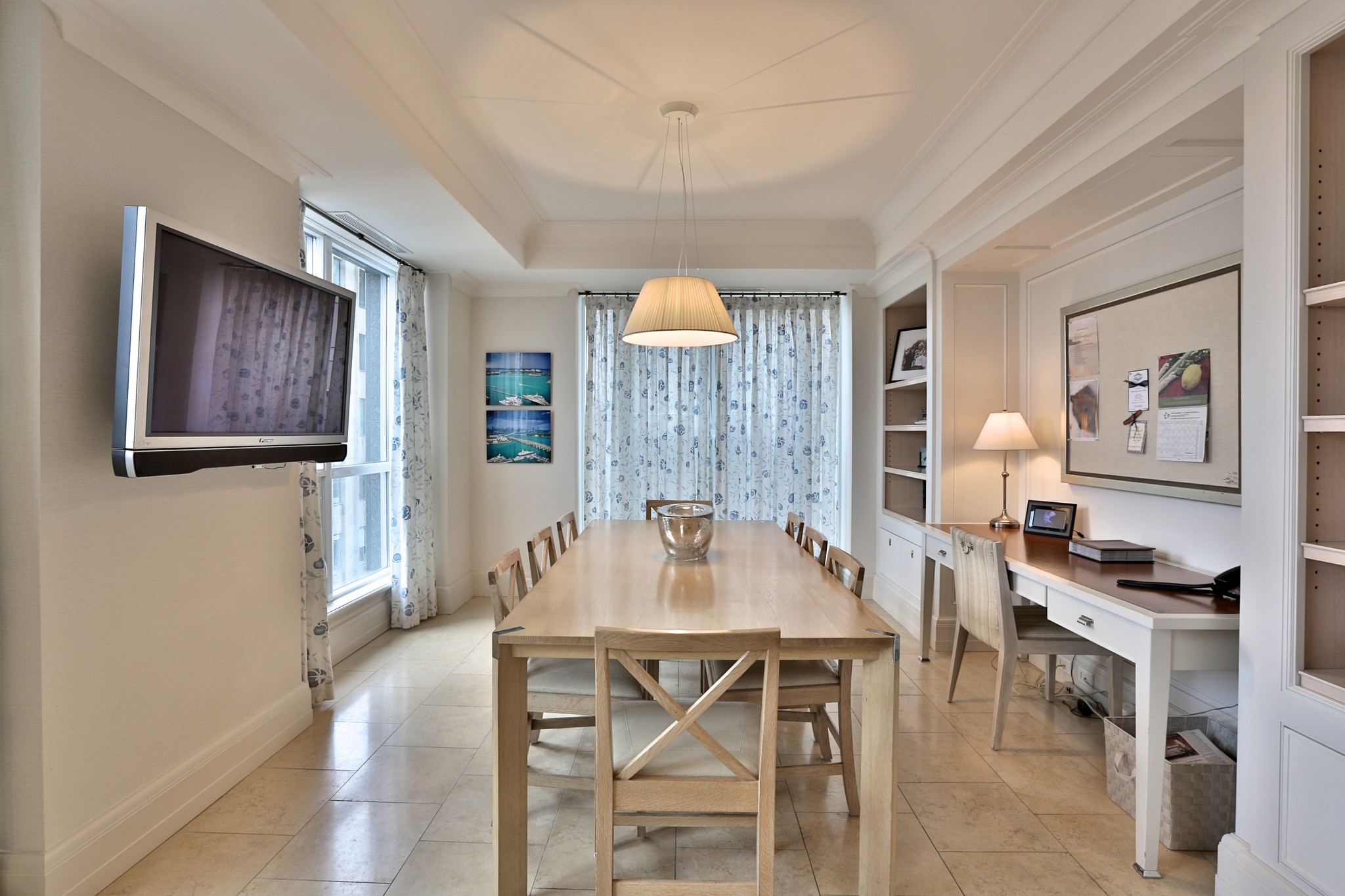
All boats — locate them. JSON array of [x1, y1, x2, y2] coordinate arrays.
[[499, 397, 522, 406], [487, 454, 507, 463], [523, 392, 548, 405], [518, 449, 534, 456], [513, 456, 525, 461]]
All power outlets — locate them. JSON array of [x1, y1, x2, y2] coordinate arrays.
[[1056, 655, 1072, 675], [1077, 666, 1094, 688]]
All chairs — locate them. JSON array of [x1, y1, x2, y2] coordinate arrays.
[[945, 526, 1122, 754], [486, 512, 867, 896]]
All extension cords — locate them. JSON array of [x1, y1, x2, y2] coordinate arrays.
[[1062, 681, 1096, 712]]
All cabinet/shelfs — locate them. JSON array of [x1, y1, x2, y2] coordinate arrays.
[[884, 375, 927, 481], [878, 528, 922, 600], [1297, 278, 1345, 566], [1281, 725, 1345, 896]]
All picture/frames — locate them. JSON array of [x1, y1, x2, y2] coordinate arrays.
[[889, 325, 927, 384]]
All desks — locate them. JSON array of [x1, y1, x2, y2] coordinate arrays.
[[916, 524, 1239, 877], [491, 519, 902, 896]]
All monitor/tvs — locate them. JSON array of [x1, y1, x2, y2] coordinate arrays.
[[110, 205, 357, 479]]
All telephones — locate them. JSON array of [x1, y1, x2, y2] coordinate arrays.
[[1212, 565, 1240, 601]]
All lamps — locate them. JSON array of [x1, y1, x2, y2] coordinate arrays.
[[972, 410, 1041, 528], [621, 101, 738, 347]]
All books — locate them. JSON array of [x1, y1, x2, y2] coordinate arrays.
[[1068, 539, 1156, 564]]
